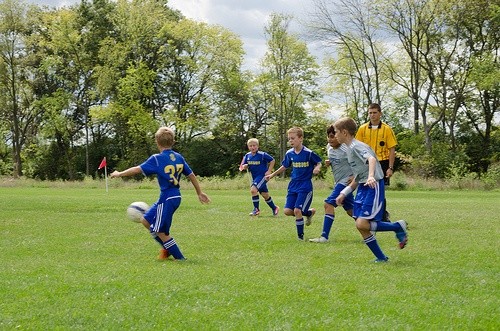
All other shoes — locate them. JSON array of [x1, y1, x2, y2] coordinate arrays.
[[370, 231, 376, 237], [309, 236, 328, 243], [157, 248, 171, 259], [249, 208, 260, 216], [273, 205, 279, 216], [305, 208, 316, 226], [385, 211, 391, 222]]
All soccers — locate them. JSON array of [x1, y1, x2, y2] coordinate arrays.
[[127, 201, 150, 224]]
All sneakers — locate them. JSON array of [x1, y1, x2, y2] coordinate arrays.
[[396, 220, 409, 249]]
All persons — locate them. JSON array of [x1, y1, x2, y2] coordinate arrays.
[[309, 125, 377, 243], [238, 138, 280, 217], [108, 126, 211, 259], [264, 127, 322, 242], [354, 103, 398, 223], [332, 117, 408, 263]]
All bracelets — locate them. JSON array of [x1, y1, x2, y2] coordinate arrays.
[[388, 167, 394, 171]]
